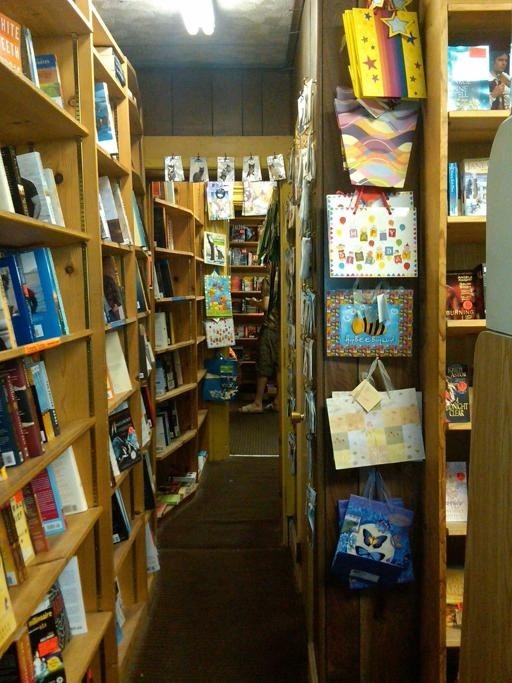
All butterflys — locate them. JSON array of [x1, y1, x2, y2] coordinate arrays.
[[354, 529, 388, 562]]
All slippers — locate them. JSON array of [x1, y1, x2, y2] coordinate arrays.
[[238, 406, 264, 415]]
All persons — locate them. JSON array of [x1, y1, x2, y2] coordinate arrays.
[[238, 250, 281, 413]]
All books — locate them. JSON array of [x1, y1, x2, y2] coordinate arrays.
[[156, 154, 286, 350], [449, 44, 512, 113], [198, 450, 208, 480], [1, 13, 161, 681], [286, 75, 316, 548], [154, 351, 198, 516], [445, 566, 466, 648], [446, 157, 489, 521]]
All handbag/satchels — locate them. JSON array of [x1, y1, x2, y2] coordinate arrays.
[[330, 468, 414, 589], [332, 0, 427, 186], [329, 187, 418, 278], [326, 360, 425, 469], [325, 278, 413, 357]]
